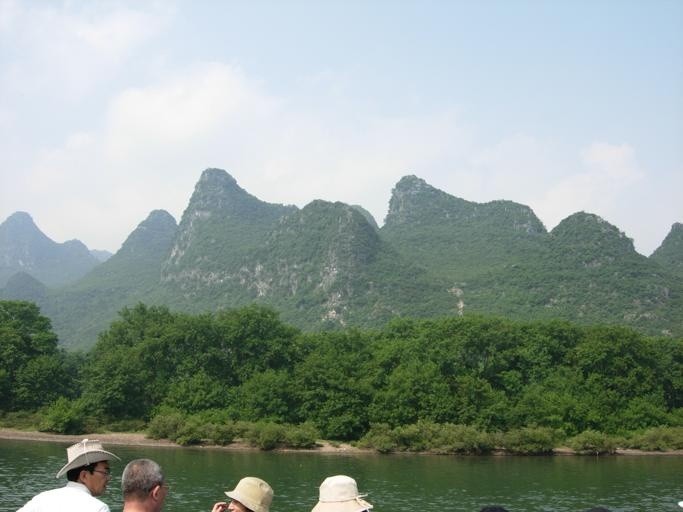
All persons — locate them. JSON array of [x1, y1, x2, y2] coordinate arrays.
[[121, 459, 169, 512], [211, 476, 274, 512], [15, 440, 112, 512]]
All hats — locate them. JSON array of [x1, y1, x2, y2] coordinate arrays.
[[224, 476, 273, 512], [311, 475, 373, 512], [55, 440, 122, 478]]
